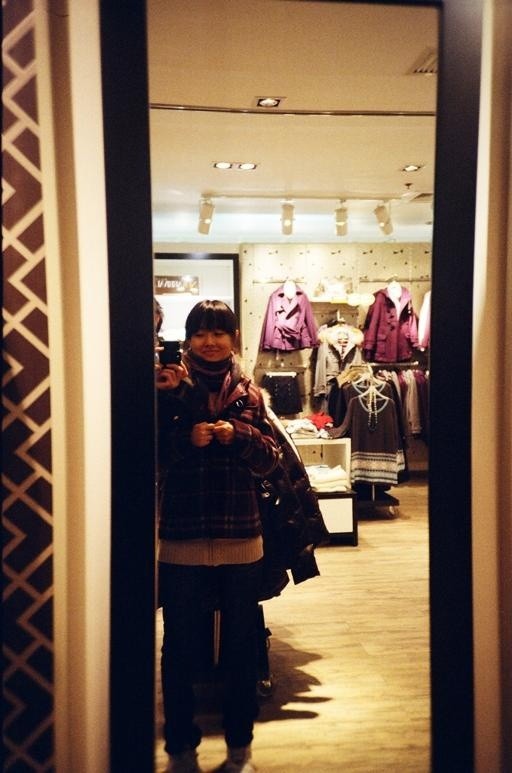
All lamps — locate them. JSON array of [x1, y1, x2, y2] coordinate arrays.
[[196, 193, 395, 237]]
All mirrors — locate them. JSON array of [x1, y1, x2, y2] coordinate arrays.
[[98, 0, 477, 773], [151, 251, 244, 342]]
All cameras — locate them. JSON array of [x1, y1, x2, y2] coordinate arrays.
[[158, 341, 182, 367]]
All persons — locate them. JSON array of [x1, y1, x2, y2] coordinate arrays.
[[154, 295, 280, 773]]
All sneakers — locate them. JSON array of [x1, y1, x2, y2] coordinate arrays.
[[224, 745, 254, 773], [162, 751, 203, 772]]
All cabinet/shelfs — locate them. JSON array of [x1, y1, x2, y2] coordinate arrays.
[[288, 432, 359, 547]]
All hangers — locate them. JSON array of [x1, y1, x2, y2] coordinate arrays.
[[329, 315, 352, 328], [337, 361, 418, 407], [264, 361, 297, 381]]
